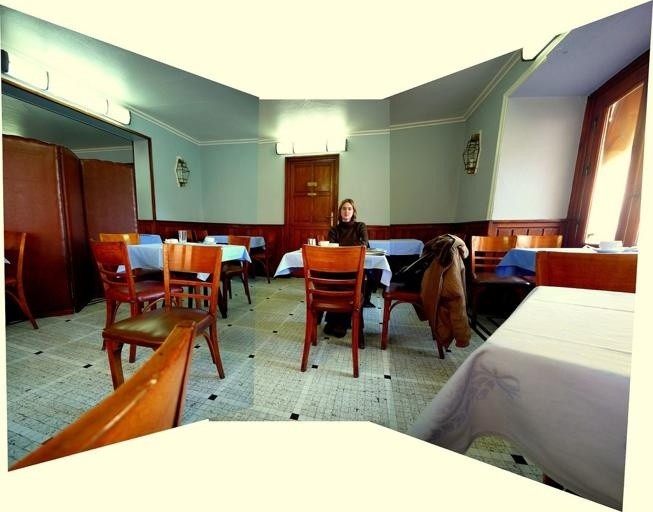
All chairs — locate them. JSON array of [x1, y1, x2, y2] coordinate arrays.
[[4, 230, 39, 329], [471, 234, 638, 328], [380, 233, 472, 359], [8, 318, 198, 471], [88, 228, 271, 390], [301, 243, 366, 378]]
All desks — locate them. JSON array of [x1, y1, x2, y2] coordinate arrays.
[[6, 419, 622, 512]]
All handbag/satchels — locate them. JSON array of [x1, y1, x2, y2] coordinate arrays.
[[390, 250, 435, 295]]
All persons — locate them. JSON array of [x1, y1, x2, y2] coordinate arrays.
[[323, 199, 368, 338]]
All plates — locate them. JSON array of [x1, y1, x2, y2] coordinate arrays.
[[591, 247, 627, 254]]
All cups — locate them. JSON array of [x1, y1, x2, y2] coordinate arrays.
[[204, 237, 216, 243], [599, 240, 623, 249], [318, 241, 339, 247], [307, 238, 316, 245], [165, 238, 178, 244], [177, 230, 188, 244]]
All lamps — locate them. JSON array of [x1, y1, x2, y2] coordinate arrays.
[[176, 159, 190, 187], [275, 138, 347, 155], [462, 130, 481, 175], [1, 49, 132, 125]]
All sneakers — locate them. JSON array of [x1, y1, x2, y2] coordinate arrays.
[[334, 326, 346, 338], [323, 322, 335, 335]]
